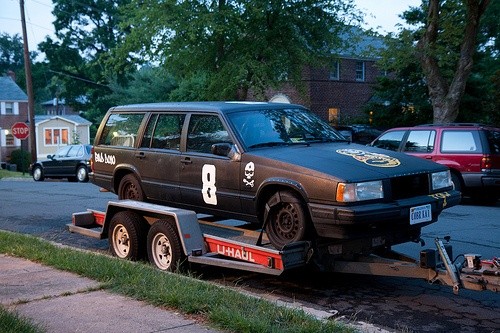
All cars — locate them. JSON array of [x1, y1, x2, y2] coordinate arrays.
[[90, 101, 462, 253], [333, 124, 383, 144], [366, 123, 500, 199], [30, 145, 91, 182]]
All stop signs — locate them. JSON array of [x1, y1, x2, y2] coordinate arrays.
[[12, 122, 31, 140]]
